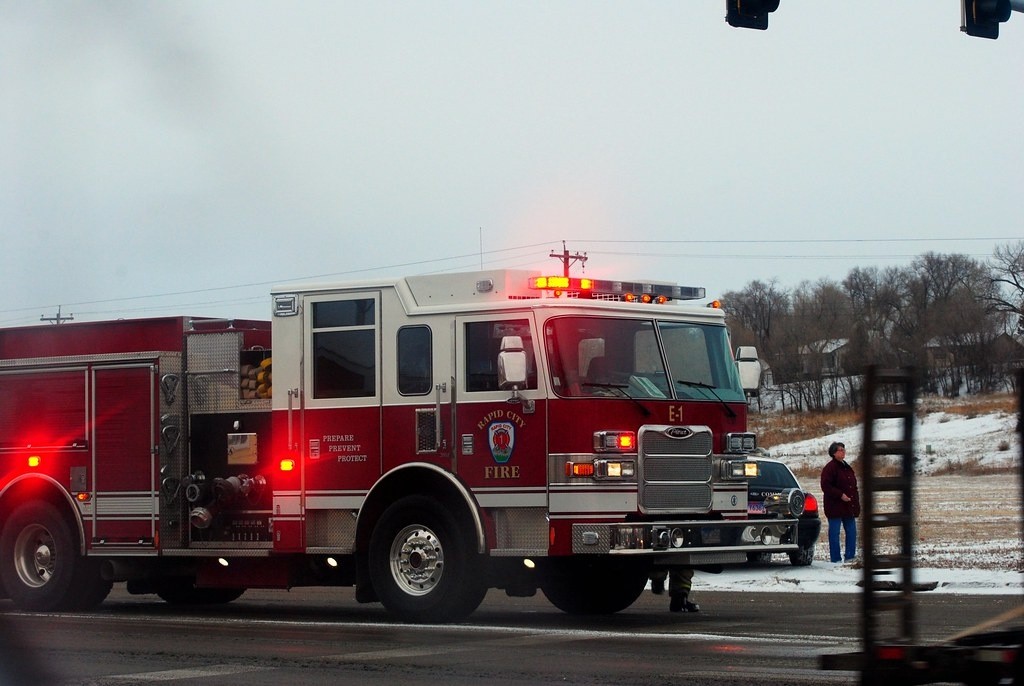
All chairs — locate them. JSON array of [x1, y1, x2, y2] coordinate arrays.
[[584, 357, 618, 394]]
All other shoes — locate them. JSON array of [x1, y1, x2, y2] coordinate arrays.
[[651, 581, 663, 592], [830, 557, 856, 563], [669, 596, 700, 612]]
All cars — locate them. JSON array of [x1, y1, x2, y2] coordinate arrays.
[[711, 455, 821, 571]]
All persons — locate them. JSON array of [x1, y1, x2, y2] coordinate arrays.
[[650, 566, 699, 612], [821, 442, 860, 563]]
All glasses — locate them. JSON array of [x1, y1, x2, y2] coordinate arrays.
[[837, 448, 846, 452]]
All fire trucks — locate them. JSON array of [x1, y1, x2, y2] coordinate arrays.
[[0, 269, 806, 625]]
